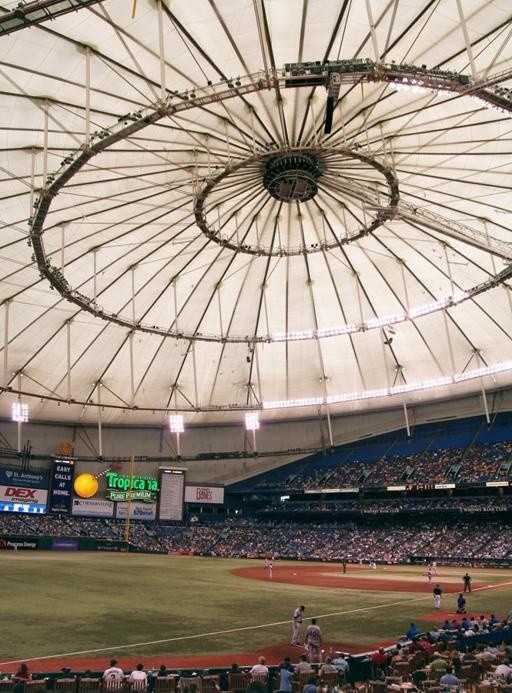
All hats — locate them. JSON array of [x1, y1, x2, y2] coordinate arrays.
[[301, 606, 304, 611], [259, 657, 265, 664], [433, 652, 440, 656]]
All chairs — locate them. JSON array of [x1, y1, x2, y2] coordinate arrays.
[[0, 639, 502, 693]]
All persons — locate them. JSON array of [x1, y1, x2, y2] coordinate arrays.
[[456, 592, 466, 614], [196, 643, 395, 693], [427, 568, 432, 584], [242, 439, 511, 569], [268, 563, 273, 578], [431, 561, 437, 576], [11, 662, 32, 692], [396, 613, 512, 693], [264, 560, 269, 574], [151, 664, 169, 692], [128, 663, 149, 692], [462, 572, 472, 592], [290, 605, 305, 647], [432, 583, 443, 610], [303, 618, 324, 662], [102, 659, 128, 692], [342, 558, 346, 574], [0, 509, 242, 558]]
